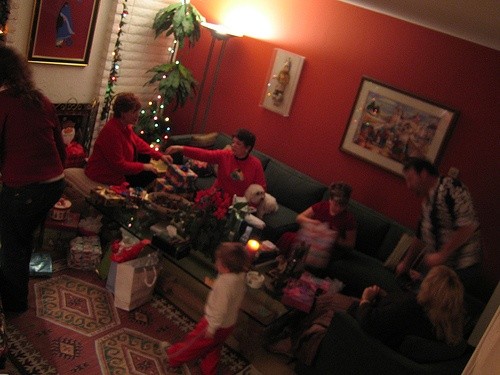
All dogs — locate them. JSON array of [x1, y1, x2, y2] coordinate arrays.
[[244, 184, 280, 219]]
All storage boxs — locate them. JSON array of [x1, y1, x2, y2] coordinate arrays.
[[29, 252, 54, 276], [285, 279, 321, 313], [90, 186, 121, 208]]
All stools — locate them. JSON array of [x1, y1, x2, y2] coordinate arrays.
[[64, 167, 107, 212], [37, 211, 83, 247]]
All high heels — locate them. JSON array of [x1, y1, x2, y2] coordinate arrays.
[[261, 343, 297, 365]]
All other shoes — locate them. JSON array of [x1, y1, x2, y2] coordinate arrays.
[[160, 341, 171, 356]]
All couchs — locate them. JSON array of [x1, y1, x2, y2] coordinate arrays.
[[290, 265, 476, 375], [162, 135, 414, 285]]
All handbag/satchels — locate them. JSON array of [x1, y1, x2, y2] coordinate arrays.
[[98, 246, 112, 280], [113, 252, 163, 313], [105, 254, 144, 293]]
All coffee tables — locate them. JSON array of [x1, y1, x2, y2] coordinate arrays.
[[79, 201, 346, 352]]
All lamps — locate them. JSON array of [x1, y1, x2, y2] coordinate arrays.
[[245, 240, 259, 256], [185, 22, 241, 134]]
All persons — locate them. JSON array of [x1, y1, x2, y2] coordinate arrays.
[[402, 155, 481, 269], [161, 241, 250, 375], [276, 182, 356, 251], [0, 44, 67, 319], [84, 92, 173, 186], [167, 129, 267, 199], [262, 267, 465, 365], [352, 98, 439, 162]]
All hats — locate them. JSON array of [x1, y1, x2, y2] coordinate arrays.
[[331, 184, 353, 206]]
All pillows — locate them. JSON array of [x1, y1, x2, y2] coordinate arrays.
[[400, 336, 466, 365]]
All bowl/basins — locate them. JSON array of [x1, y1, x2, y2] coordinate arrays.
[[145, 192, 190, 216]]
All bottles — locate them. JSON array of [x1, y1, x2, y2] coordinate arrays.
[[282, 241, 312, 280]]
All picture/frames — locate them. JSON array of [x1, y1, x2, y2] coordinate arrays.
[[339, 77, 460, 182], [55, 101, 101, 152], [27, 0, 103, 67]]
